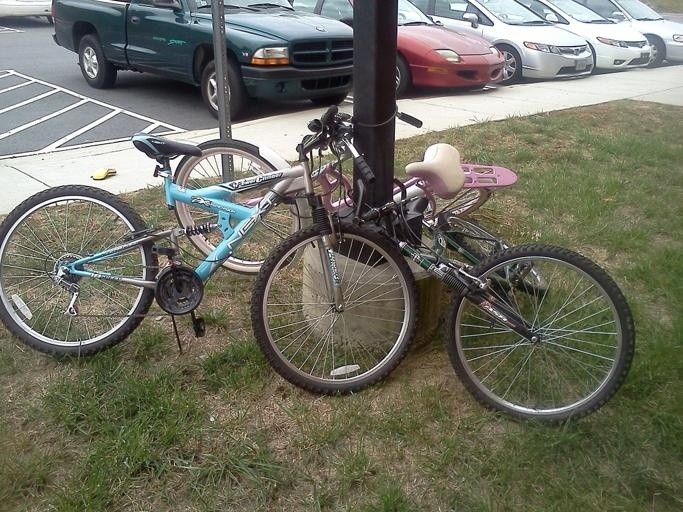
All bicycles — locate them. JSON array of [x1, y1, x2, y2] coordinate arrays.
[[1, 105, 421, 399], [172, 109, 517, 276], [354, 176, 637, 426]]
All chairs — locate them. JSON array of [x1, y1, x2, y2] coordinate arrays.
[[433, 1, 451, 18]]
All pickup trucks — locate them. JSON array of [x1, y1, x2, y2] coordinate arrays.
[[51, 0, 353, 117]]
